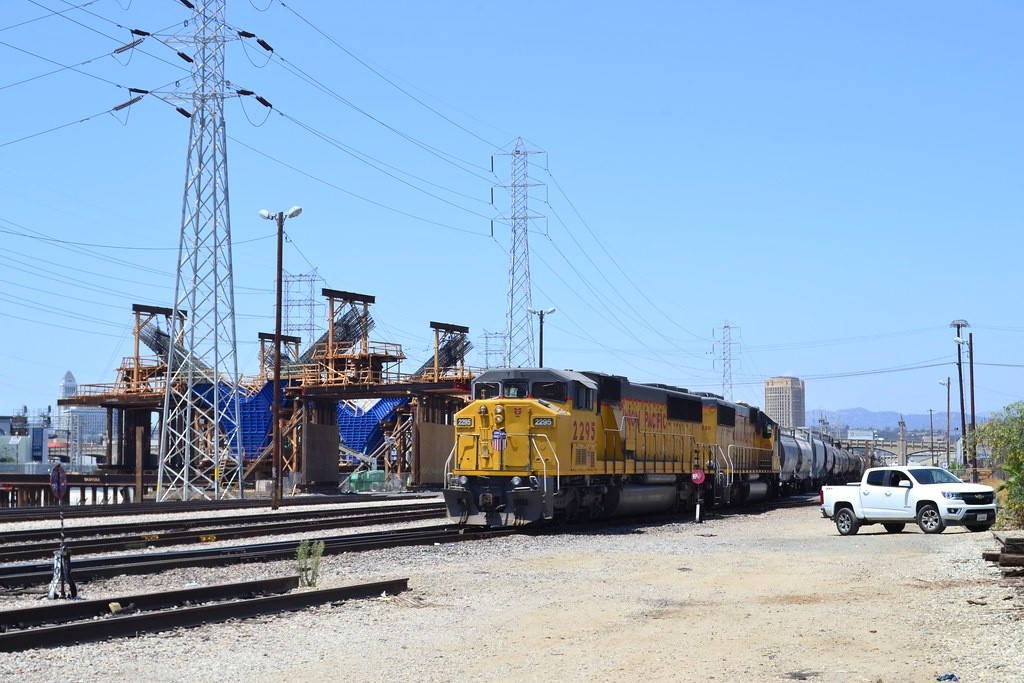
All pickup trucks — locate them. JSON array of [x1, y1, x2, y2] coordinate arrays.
[[818, 464, 999, 538]]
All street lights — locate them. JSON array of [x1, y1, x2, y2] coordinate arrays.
[[939, 376, 951, 467], [926, 408, 935, 466], [953, 332, 978, 482], [258, 205, 303, 511], [948, 319, 970, 482], [527, 306, 556, 369]]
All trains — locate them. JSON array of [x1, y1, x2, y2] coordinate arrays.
[[437, 364, 864, 534]]
